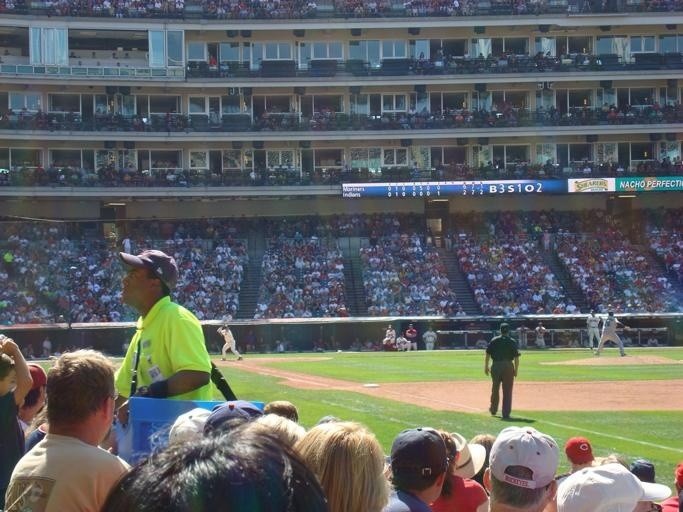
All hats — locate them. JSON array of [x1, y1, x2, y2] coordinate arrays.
[[203, 400, 264, 438], [120, 250, 179, 292], [489, 426, 561, 488], [27, 363, 48, 389], [449, 431, 487, 480], [565, 436, 595, 465], [169, 408, 212, 444], [674, 463, 683, 488], [629, 459, 656, 483], [557, 462, 672, 512], [389, 425, 447, 480]]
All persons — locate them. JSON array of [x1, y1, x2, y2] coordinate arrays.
[[1, 97, 682, 132], [1, 0, 683, 17], [216, 322, 243, 361], [485, 325, 519, 419], [112, 249, 211, 428], [410, 49, 602, 71], [102, 399, 683, 511], [1, 207, 682, 356], [2, 355, 132, 510], [1, 155, 682, 186], [70, 52, 131, 59], [208, 55, 229, 78]]
